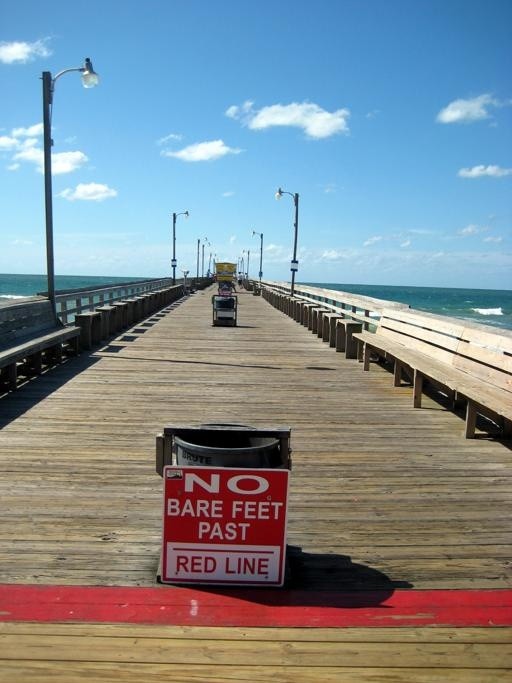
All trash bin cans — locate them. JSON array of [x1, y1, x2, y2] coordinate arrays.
[[212, 295, 237, 327], [156, 420, 294, 587]]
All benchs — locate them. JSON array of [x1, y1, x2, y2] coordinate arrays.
[[352, 307, 511, 439], [1, 300, 81, 391]]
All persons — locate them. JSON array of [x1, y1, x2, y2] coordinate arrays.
[[237, 271, 243, 289]]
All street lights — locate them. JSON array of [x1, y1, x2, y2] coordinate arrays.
[[42, 57, 100, 326], [172, 210, 188, 285], [251, 230, 263, 282], [197, 236, 219, 281], [238, 249, 249, 279], [275, 186, 299, 297]]
